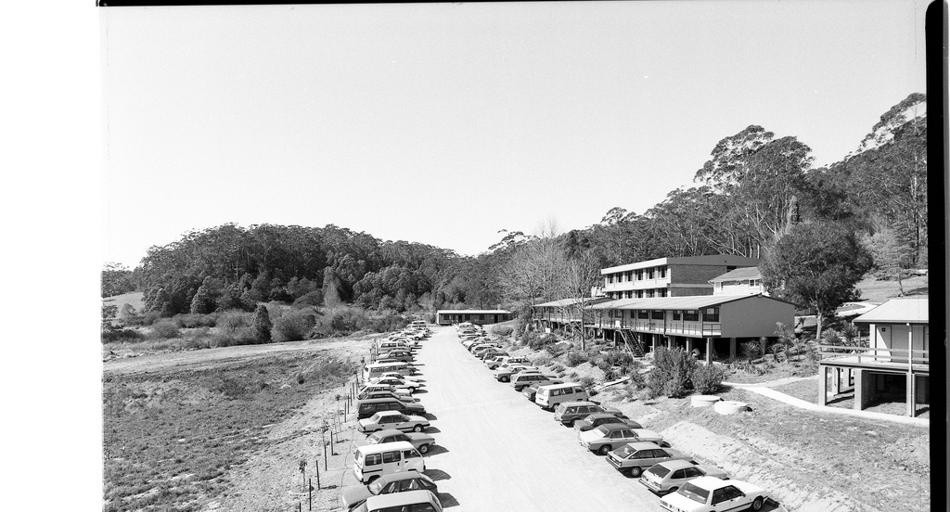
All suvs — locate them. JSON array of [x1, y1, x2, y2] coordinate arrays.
[[554, 401, 623, 425]]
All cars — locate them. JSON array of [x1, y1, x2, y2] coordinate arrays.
[[574, 413, 643, 434], [660, 476, 769, 512], [342, 471, 439, 509], [457, 322, 590, 410], [638, 459, 727, 496], [577, 423, 664, 455], [347, 489, 443, 512], [605, 441, 693, 476], [352, 429, 435, 455], [358, 410, 431, 434], [343, 320, 443, 418]]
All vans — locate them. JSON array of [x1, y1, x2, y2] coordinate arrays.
[[353, 441, 426, 485]]
